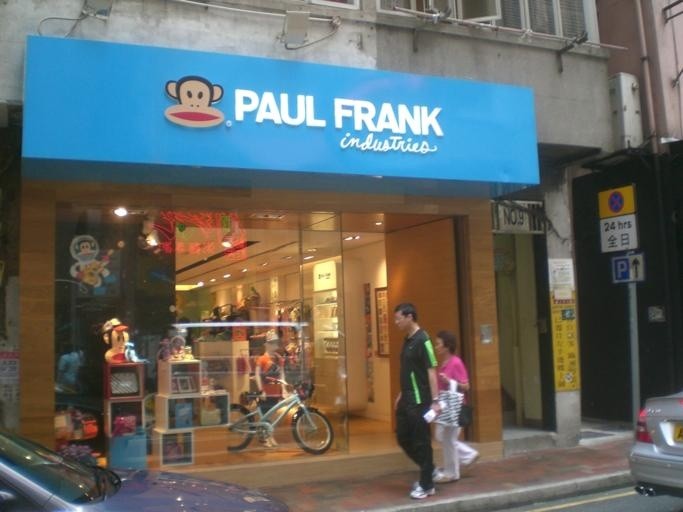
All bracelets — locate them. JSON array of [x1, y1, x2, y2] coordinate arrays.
[[433, 397, 439, 403], [260, 387, 265, 394]]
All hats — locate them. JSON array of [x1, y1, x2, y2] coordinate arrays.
[[102, 318, 128, 332], [265, 329, 279, 341]]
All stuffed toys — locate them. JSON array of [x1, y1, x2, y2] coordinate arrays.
[[99, 317, 130, 364], [157, 335, 196, 363]]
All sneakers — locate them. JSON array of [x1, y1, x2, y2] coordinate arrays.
[[412, 469, 442, 489], [465, 451, 479, 466], [433, 472, 450, 483], [410, 487, 435, 499]]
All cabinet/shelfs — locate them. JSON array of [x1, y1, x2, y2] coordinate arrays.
[[102, 357, 231, 469], [311, 288, 338, 359]]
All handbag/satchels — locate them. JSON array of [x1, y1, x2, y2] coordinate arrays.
[[459, 405, 473, 426], [434, 379, 464, 427], [114, 416, 135, 433]]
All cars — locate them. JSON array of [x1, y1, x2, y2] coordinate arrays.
[[0, 430, 289, 512], [626, 392, 683, 497]]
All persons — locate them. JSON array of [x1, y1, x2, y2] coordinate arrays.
[[393, 301, 444, 501], [432, 330, 481, 485], [56, 349, 100, 388], [255, 329, 289, 448]]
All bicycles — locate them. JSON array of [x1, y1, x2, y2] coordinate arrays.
[[227, 377, 334, 454]]
